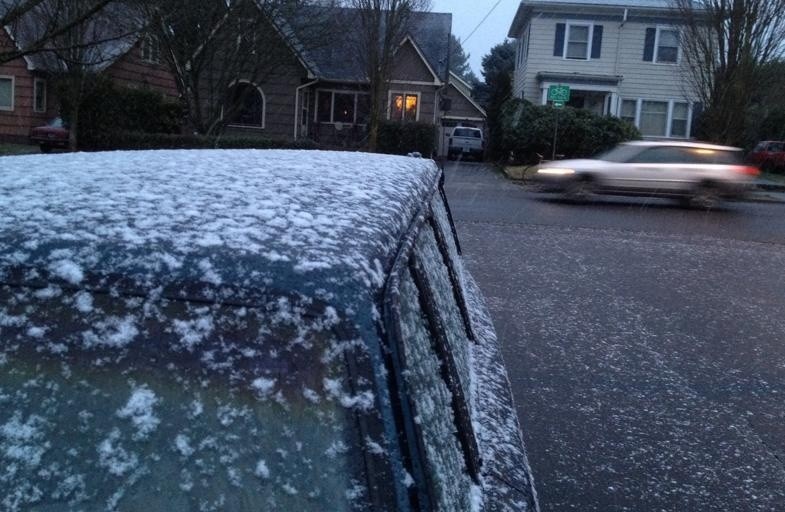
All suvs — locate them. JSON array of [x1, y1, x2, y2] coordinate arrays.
[[533, 140, 761, 211]]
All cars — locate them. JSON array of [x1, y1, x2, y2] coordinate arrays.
[[748, 140, 785, 171]]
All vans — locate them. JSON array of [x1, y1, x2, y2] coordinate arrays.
[[447, 126, 485, 164], [1, 147, 544, 511]]
